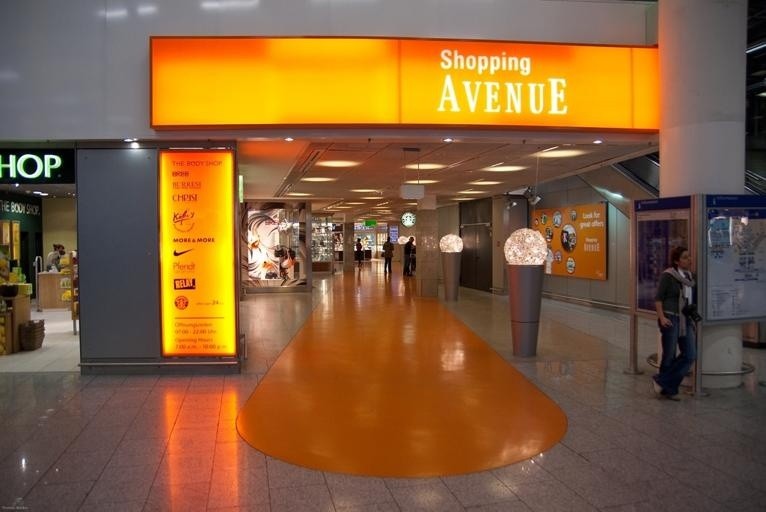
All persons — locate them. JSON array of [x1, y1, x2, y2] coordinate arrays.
[[403, 237, 414, 276], [652, 245, 698, 403], [46, 244, 64, 272], [383, 236, 394, 272], [356, 238, 362, 267]]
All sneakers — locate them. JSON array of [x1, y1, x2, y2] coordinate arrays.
[[652, 374, 682, 402]]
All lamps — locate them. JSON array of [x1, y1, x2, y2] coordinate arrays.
[[523, 146, 542, 205], [502, 191, 517, 211]]
